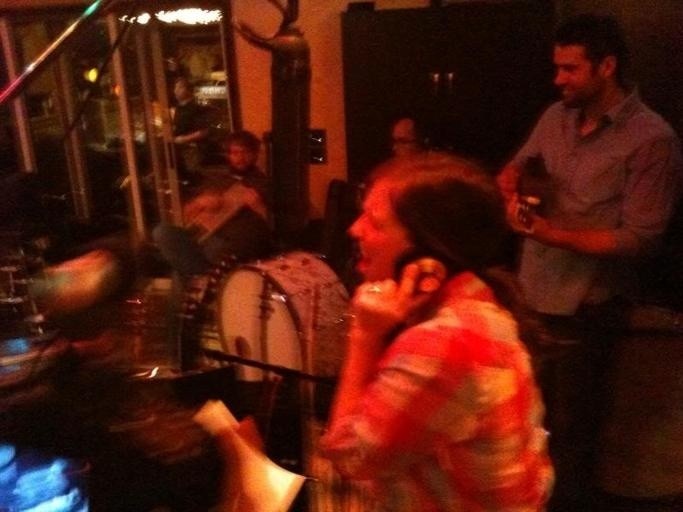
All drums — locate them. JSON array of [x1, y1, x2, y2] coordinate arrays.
[[216, 251, 352, 384]]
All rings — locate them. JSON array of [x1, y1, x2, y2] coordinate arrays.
[[366, 286, 381, 294]]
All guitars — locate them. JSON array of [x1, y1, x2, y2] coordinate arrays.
[[516, 174, 545, 229]]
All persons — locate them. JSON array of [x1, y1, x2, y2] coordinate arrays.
[[495, 14, 679, 493], [311, 149, 554, 512], [166, 76, 219, 169], [184, 128, 278, 227], [391, 111, 427, 154]]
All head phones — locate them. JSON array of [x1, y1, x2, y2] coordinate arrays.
[[391, 244, 451, 294]]
